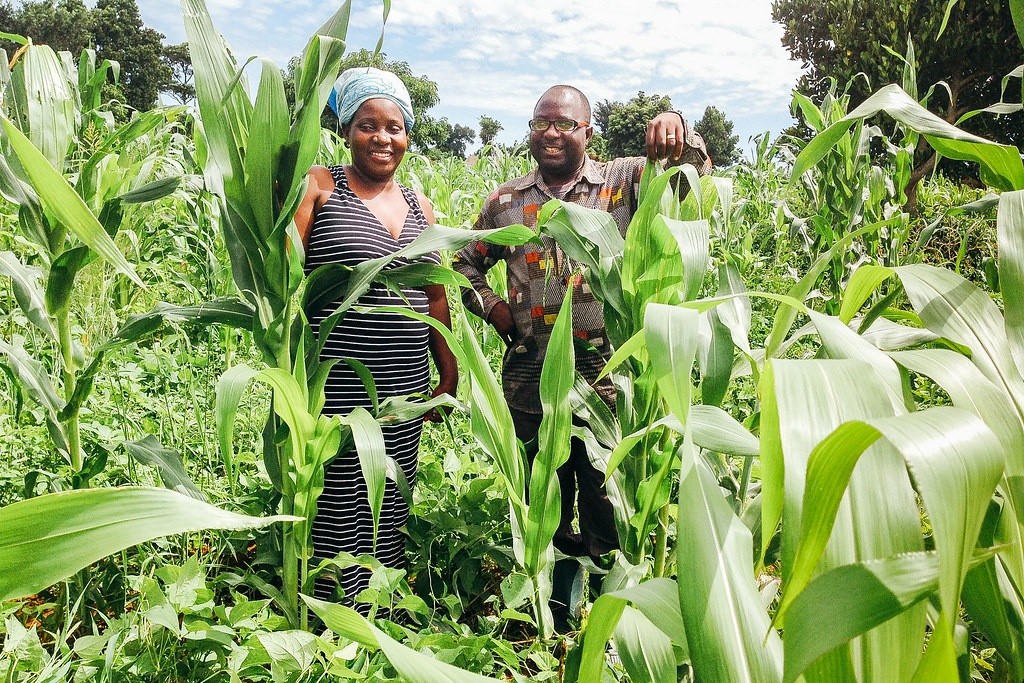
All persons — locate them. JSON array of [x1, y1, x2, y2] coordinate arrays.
[[453, 85, 713, 657], [286, 67, 459, 637]]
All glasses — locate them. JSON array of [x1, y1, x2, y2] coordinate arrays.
[[529, 119, 589, 131]]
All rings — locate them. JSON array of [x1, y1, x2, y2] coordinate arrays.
[[667, 135, 675, 139]]
